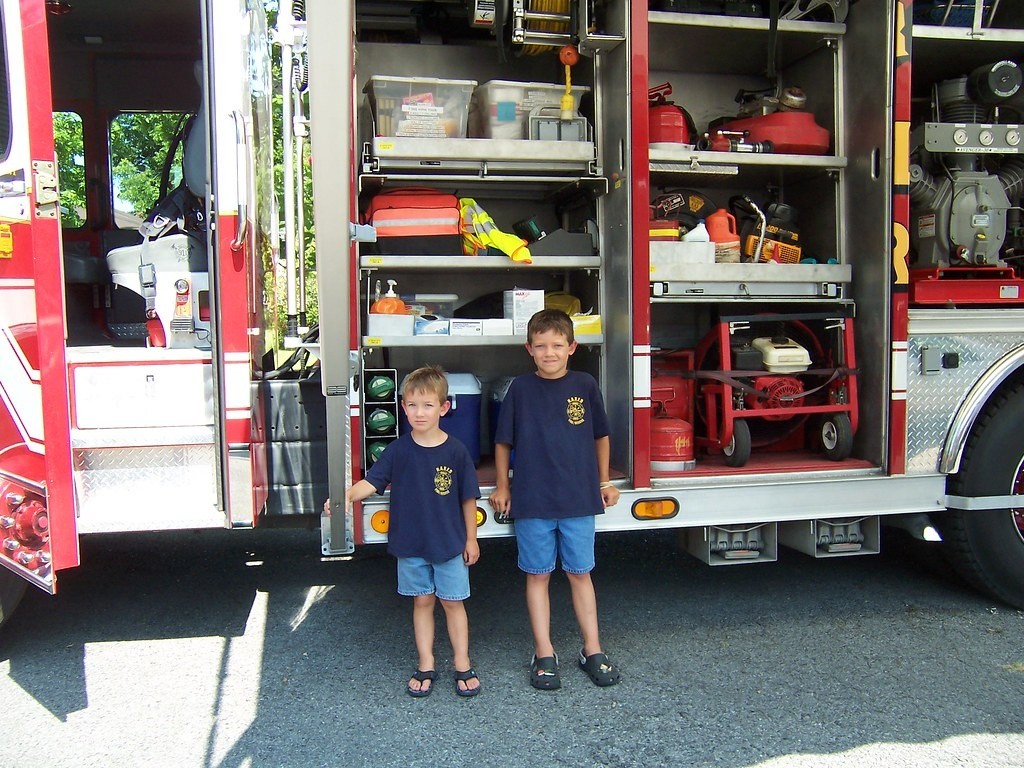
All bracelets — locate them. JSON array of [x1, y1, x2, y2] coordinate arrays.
[[600, 481, 613, 489]]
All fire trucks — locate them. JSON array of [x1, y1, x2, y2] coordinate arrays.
[[0, 0, 1024, 621]]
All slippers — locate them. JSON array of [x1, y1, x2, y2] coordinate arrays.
[[408, 667, 438, 697], [454, 668, 481, 696]]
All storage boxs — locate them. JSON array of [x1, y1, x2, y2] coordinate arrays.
[[481, 318, 514, 336], [447, 318, 482, 335], [367, 314, 415, 336], [487, 375, 515, 470], [362, 75, 479, 137], [569, 314, 602, 334], [503, 289, 545, 336], [398, 371, 483, 470], [415, 321, 450, 336], [468, 80, 590, 140]]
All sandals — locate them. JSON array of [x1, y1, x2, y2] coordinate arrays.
[[578, 648, 619, 686], [530, 651, 560, 690]]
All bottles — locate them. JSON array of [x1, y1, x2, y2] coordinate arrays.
[[681, 218, 710, 242], [705, 208, 741, 263]]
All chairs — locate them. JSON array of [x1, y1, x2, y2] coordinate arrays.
[[106, 59, 205, 274]]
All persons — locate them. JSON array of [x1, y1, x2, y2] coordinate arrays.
[[488, 309, 621, 689], [323, 368, 481, 697]]
